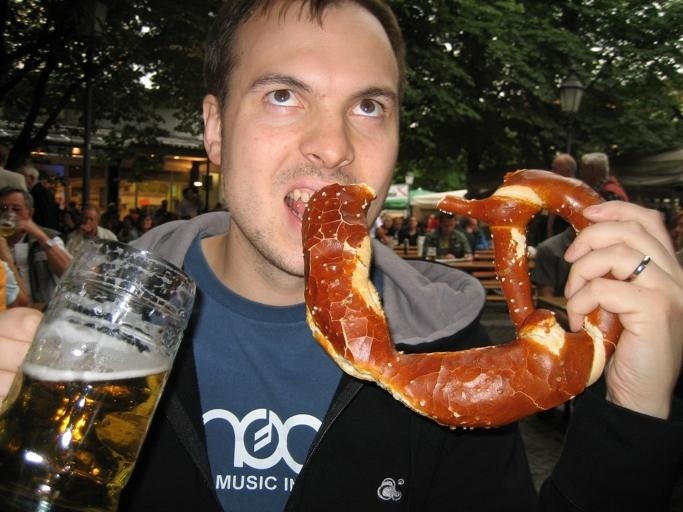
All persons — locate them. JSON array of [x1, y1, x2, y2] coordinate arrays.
[[368, 213, 490, 262], [0, 165, 229, 313], [0, 0, 683, 512], [528, 151, 683, 299]]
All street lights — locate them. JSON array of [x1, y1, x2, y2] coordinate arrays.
[[560, 73, 584, 154]]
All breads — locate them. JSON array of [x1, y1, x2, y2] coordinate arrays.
[[301, 168, 623, 430]]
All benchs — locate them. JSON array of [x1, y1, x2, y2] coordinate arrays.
[[390, 241, 574, 421]]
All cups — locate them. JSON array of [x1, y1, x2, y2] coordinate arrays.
[[0, 237, 198, 512]]
